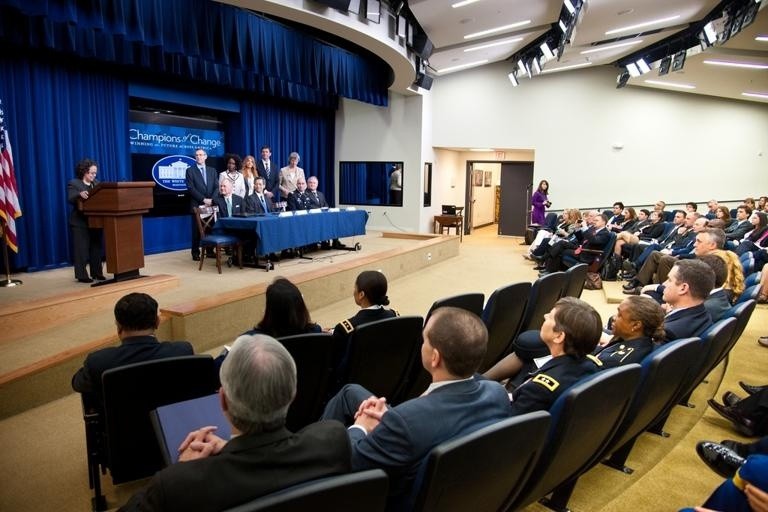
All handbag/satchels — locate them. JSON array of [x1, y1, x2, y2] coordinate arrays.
[[585, 272, 601, 290]]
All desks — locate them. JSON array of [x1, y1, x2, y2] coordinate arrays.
[[215, 208, 370, 271]]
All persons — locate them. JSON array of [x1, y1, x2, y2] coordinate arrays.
[[481, 295, 665, 381], [531, 180, 768, 347], [319, 307, 511, 498], [71, 293, 193, 393], [480, 296, 606, 418], [115, 332, 352, 512], [66, 158, 105, 283], [334, 271, 400, 336], [186, 146, 346, 261], [390, 164, 403, 205], [678, 380, 768, 512], [239, 277, 322, 337]]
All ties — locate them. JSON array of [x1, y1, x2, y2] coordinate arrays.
[[266, 163, 270, 179], [201, 168, 207, 186], [260, 196, 268, 214], [226, 197, 234, 217]]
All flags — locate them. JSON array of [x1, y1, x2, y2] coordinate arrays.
[[0, 98, 23, 254]]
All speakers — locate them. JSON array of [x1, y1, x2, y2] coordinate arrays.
[[417, 72, 433, 91], [412, 33, 433, 60]]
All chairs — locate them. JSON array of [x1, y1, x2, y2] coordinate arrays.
[[193, 205, 241, 274], [443, 207, 464, 234]]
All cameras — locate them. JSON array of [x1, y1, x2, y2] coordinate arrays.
[[546, 201, 552, 208]]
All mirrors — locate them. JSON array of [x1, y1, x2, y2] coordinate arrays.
[[339, 161, 404, 207]]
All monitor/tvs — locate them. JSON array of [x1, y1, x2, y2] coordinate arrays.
[[442, 205, 456, 216], [348, 0, 361, 16], [614, 0, 760, 88], [407, 24, 413, 47], [365, 0, 381, 24], [396, 15, 407, 38], [507, 0, 586, 88]]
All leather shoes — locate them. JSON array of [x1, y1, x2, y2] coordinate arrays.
[[78, 278, 93, 282], [207, 254, 216, 258], [193, 255, 200, 261], [523, 253, 550, 277], [270, 241, 345, 262], [696, 381, 763, 479], [623, 260, 636, 295], [93, 274, 106, 280], [758, 336, 768, 346]]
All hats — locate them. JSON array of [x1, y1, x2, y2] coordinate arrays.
[[513, 330, 550, 358]]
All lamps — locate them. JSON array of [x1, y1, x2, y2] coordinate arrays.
[[507, 0, 582, 87], [612, 0, 767, 88], [328, 0, 436, 90]]
